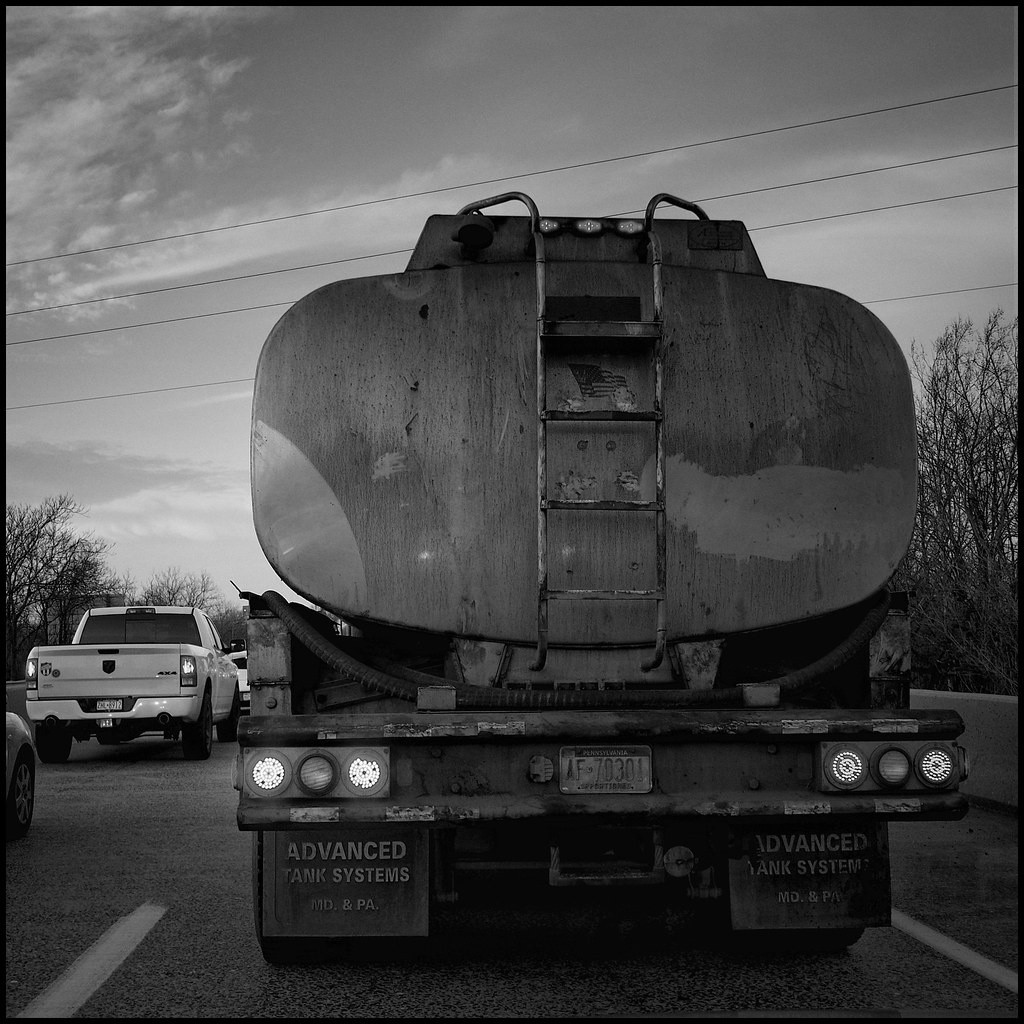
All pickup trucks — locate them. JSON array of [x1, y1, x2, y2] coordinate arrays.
[[26, 605, 240, 761]]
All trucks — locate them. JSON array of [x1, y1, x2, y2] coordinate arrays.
[[235, 189, 972, 969]]
